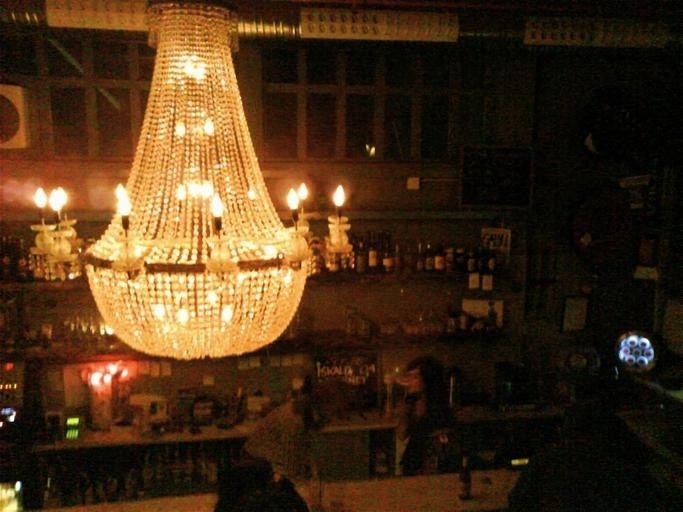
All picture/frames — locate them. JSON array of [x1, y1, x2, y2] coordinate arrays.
[[457, 144, 534, 210]]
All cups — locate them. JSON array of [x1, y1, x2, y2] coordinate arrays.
[[445, 313, 468, 333], [343, 304, 400, 336]]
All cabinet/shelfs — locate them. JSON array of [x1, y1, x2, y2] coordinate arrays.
[[1, 212, 506, 363]]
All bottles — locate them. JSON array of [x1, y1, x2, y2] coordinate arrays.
[[64, 438, 234, 507], [456, 452, 471, 498], [0, 233, 42, 281], [309, 233, 498, 272]]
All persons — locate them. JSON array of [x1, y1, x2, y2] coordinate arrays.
[[390, 352, 449, 475], [236, 382, 329, 479]]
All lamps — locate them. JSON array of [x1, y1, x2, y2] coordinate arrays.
[[28, 0, 356, 360]]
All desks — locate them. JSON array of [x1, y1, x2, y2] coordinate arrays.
[[38, 468, 521, 512], [35, 408, 400, 480]]
